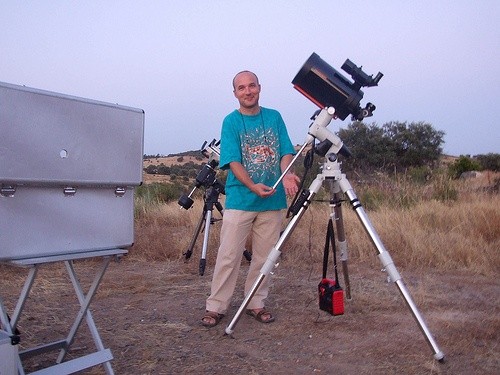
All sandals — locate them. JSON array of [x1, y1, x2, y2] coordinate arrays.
[[200, 311, 224, 327], [246, 308, 275, 323]]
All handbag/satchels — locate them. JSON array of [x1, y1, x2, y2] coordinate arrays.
[[318, 278, 345, 315]]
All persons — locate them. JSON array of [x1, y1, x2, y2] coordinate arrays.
[[202, 70, 302, 327]]
